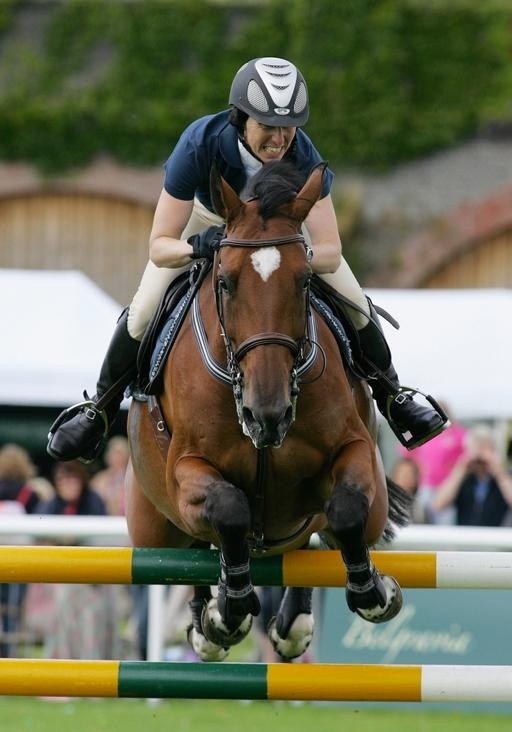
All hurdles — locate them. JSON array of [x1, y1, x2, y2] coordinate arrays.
[[0, 545, 512, 704]]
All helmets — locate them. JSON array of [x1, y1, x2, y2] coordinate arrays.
[[228, 57, 311, 129]]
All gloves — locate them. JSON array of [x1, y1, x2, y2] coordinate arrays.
[[187, 225, 214, 262]]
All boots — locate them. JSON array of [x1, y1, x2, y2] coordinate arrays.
[[334, 295, 444, 439], [46, 305, 140, 461]]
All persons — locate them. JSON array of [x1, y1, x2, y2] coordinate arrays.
[[46, 57, 451, 464], [2, 405, 512, 665]]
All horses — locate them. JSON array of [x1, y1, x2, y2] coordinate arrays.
[[126, 163, 414, 664]]
